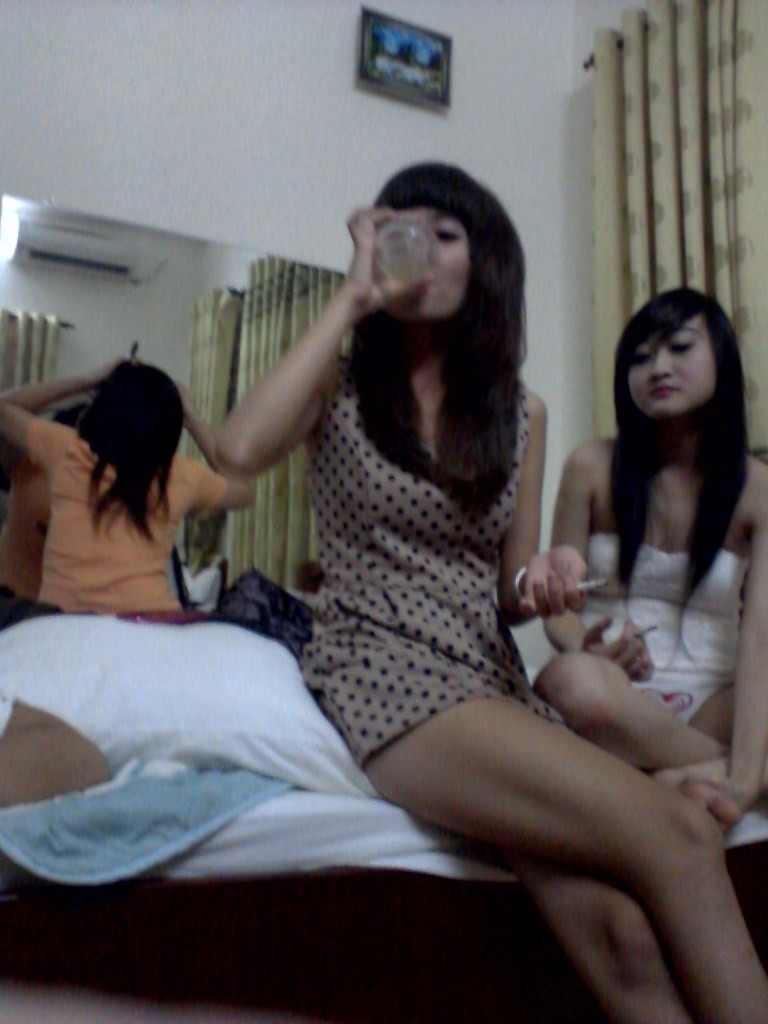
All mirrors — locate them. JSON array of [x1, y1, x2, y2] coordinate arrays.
[[1, 193, 351, 628]]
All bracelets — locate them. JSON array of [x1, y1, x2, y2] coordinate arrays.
[[513, 566, 527, 600]]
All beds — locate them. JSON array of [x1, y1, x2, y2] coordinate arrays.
[[1, 625, 768, 1024]]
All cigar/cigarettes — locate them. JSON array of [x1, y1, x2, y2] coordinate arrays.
[[576, 578, 607, 590]]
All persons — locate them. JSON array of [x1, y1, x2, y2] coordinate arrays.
[[531, 286, 768, 834], [0, 393, 93, 632], [214, 161, 768, 1024], [0, 340, 255, 632]]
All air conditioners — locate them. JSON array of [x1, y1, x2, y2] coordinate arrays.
[[11, 217, 140, 280]]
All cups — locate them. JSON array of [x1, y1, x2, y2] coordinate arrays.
[[372, 220, 435, 286]]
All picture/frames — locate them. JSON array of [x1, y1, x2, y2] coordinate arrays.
[[357, 4, 452, 110]]
